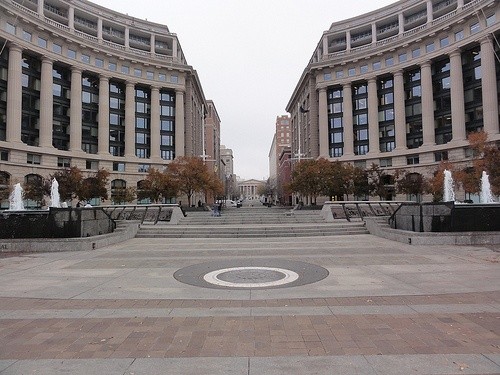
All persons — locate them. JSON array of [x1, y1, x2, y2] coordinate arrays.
[[77, 201, 83, 207]]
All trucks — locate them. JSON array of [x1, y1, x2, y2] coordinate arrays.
[[216, 200, 237, 208]]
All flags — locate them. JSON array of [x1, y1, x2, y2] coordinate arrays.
[[300, 106, 309, 113]]
